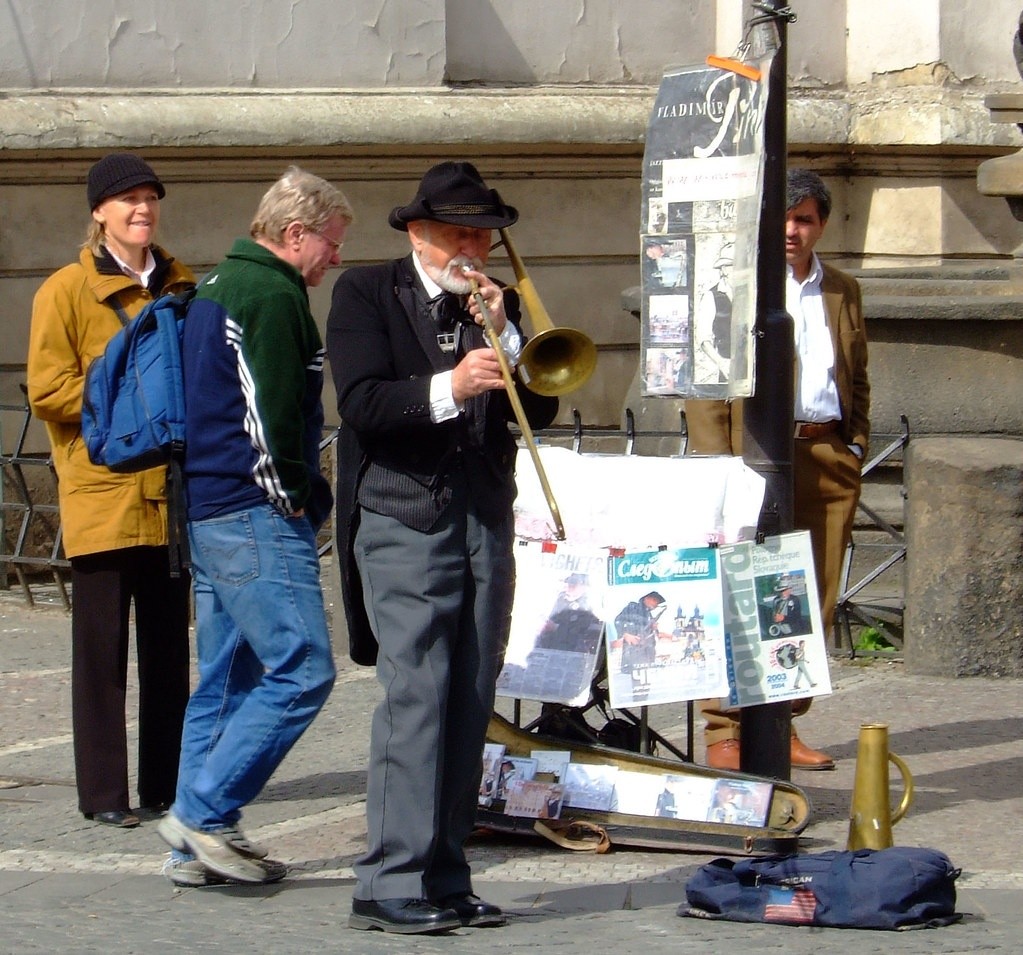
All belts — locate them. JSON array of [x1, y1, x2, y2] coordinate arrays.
[[793, 420, 839, 440]]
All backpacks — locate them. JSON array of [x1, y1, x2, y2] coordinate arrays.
[[83, 269, 219, 474]]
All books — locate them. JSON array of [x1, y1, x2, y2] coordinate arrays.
[[476, 527, 834, 830]]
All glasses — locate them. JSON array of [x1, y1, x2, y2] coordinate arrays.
[[279, 224, 343, 256]]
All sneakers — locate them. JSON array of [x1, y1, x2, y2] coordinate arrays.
[[169, 823, 269, 887], [155, 805, 287, 882]]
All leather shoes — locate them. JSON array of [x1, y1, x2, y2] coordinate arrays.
[[791, 734, 834, 770], [91, 809, 141, 828], [707, 739, 740, 769], [138, 797, 177, 812], [434, 891, 506, 926], [348, 893, 462, 933]]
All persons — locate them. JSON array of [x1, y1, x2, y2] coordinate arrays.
[[658, 776, 678, 819], [793, 640, 817, 689], [155, 165, 355, 887], [673, 349, 689, 387], [533, 573, 603, 654], [614, 591, 666, 702], [709, 780, 748, 824], [700, 168, 870, 773], [326, 162, 561, 933], [773, 580, 803, 637], [26, 154, 193, 829]]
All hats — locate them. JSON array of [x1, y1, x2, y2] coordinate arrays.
[[87, 153, 165, 212], [388, 160, 520, 233]]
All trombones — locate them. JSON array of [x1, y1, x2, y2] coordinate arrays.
[[461, 225, 598, 545]]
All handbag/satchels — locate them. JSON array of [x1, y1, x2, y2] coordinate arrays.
[[679, 847, 965, 931]]
